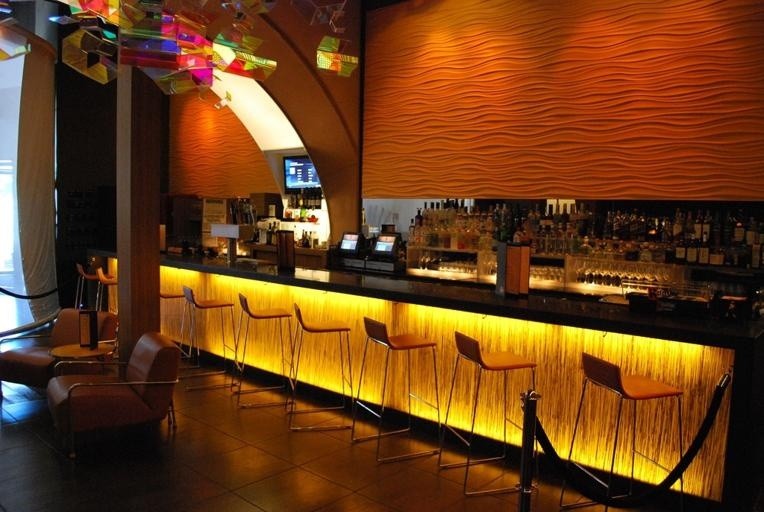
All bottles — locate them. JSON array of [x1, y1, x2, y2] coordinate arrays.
[[265, 221, 279, 246], [287, 186, 325, 209], [302, 229, 313, 248], [408, 197, 764, 287]]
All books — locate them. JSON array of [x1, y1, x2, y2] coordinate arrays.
[[494, 238, 532, 297], [273, 229, 295, 273]]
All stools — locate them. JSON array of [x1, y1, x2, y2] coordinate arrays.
[[162, 286, 351, 432], [76, 265, 118, 311], [351, 316, 441, 465], [560, 352, 683, 511], [439, 330, 536, 497]]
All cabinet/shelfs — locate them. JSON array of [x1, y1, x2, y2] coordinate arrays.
[[405, 230, 744, 302]]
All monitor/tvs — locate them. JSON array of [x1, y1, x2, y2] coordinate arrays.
[[283, 156, 322, 191], [372, 234, 400, 262], [337, 231, 362, 259]]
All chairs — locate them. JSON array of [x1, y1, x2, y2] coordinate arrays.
[[0, 307, 182, 459]]
